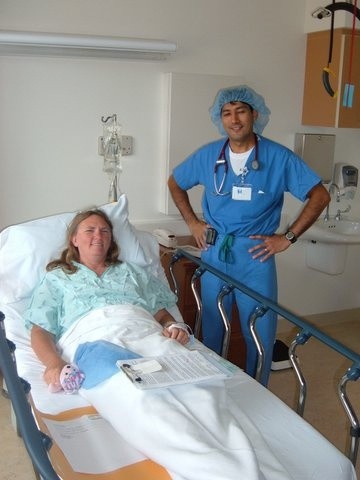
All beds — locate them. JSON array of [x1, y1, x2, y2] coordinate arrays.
[[0, 230, 360, 480]]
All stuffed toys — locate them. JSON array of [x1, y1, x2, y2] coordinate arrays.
[[39, 361, 85, 396]]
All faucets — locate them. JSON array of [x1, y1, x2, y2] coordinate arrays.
[[322, 183, 343, 222]]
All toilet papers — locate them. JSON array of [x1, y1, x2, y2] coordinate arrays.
[[331, 161, 359, 201]]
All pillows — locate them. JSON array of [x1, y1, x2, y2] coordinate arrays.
[[0, 193, 154, 305]]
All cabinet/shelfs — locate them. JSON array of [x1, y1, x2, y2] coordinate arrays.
[[300, 27, 359, 130]]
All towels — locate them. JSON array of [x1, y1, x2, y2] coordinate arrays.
[[70, 340, 143, 390]]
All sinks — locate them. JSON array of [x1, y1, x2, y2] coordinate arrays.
[[287, 212, 360, 245]]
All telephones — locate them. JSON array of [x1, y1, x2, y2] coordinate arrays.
[[153, 228, 178, 248]]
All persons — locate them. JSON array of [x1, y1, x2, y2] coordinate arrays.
[[166, 85, 332, 388], [24, 209, 190, 391]]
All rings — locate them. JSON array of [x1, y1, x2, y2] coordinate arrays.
[[264, 248, 269, 253]]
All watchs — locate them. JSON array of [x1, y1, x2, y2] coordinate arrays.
[[284, 230, 297, 244]]
